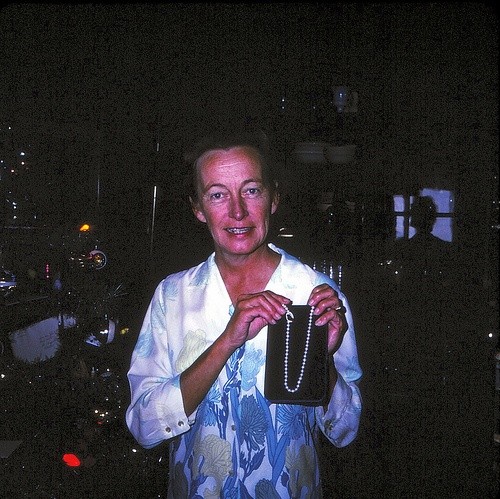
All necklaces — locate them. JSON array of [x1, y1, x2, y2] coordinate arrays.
[[281, 304, 315, 392]]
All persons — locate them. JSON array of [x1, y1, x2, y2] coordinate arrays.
[[392, 197, 455, 288], [125, 128, 363, 499]]
